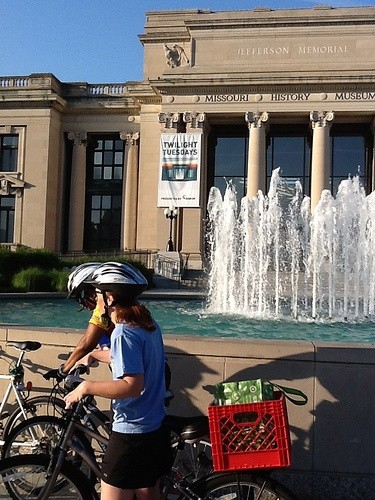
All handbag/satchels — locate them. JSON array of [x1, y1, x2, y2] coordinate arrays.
[[213, 377, 308, 405]]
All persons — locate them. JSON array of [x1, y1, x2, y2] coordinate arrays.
[[57, 260, 172, 408], [65, 261, 167, 500]]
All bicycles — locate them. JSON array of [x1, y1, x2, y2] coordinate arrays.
[[0, 341, 297, 500]]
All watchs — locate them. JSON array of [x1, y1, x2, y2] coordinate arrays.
[[59, 363, 68, 376]]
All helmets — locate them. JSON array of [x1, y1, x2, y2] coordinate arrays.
[[83, 261, 149, 306], [64, 262, 100, 301]]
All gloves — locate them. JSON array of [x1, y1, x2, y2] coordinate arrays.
[[44, 363, 67, 383]]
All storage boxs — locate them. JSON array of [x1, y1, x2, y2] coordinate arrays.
[[207, 390, 292, 473]]
